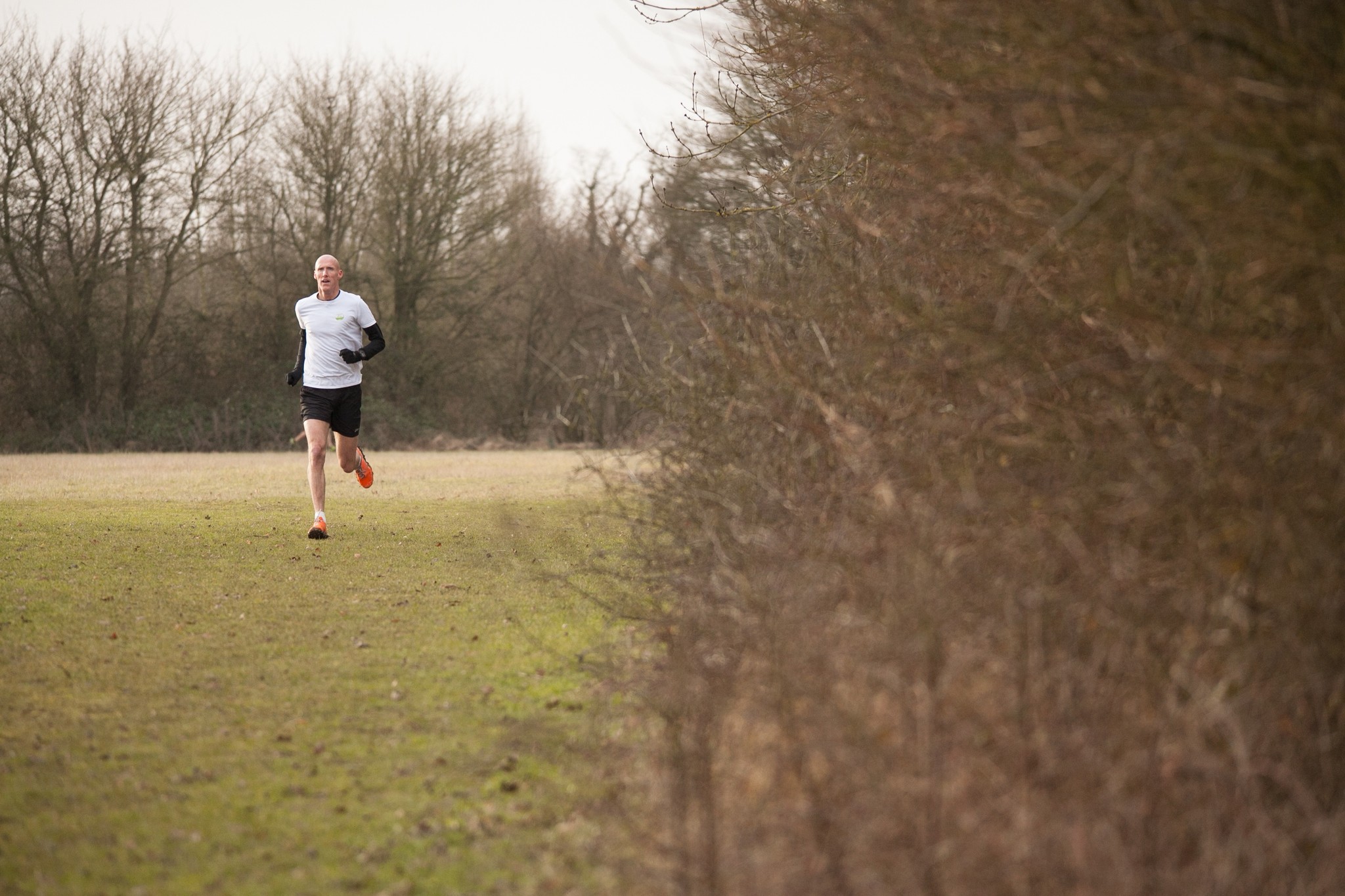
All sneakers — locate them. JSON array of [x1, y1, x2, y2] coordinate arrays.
[[308, 517, 329, 539], [354, 446, 373, 489]]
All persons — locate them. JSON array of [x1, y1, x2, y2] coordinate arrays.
[[285, 255, 386, 541]]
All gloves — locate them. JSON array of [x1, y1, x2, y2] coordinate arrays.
[[339, 349, 361, 364], [286, 370, 301, 387]]
